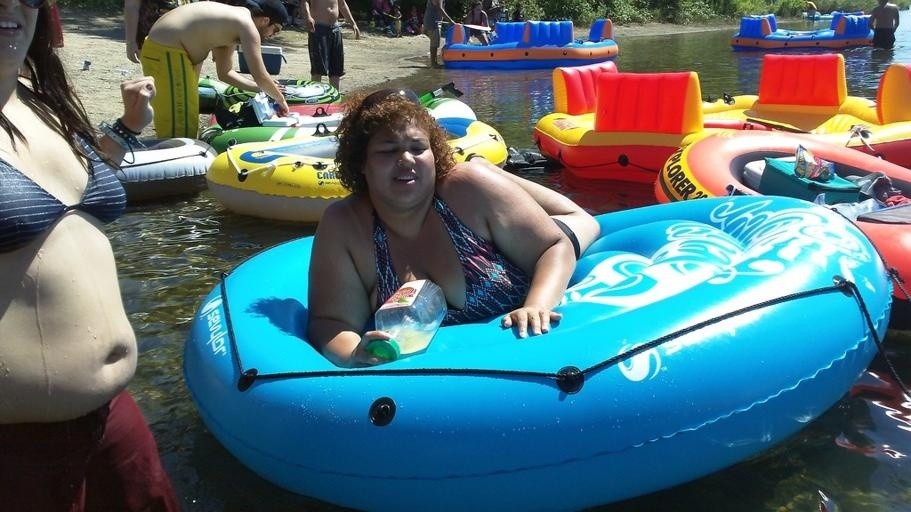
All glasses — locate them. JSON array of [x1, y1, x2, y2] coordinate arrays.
[[20, 1, 45, 8], [352, 88, 420, 127], [272, 26, 280, 37]]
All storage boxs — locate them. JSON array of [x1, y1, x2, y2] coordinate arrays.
[[236, 43, 284, 74], [758, 157, 859, 207]]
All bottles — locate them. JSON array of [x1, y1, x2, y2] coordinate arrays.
[[367, 280, 447, 362]]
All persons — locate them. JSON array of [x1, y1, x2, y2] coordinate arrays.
[[367, 1, 525, 68], [868, 0, 899, 49], [306, 88, 602, 369], [124, 1, 215, 84], [804, 1, 817, 23], [871, 49, 894, 75], [136, 0, 292, 145], [288, 1, 302, 28], [0, 0, 179, 510], [303, 1, 361, 92]]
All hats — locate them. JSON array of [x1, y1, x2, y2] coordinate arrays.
[[246, 0, 287, 22]]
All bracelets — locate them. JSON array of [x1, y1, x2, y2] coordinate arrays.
[[97, 117, 147, 155]]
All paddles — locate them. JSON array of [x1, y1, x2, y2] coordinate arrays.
[[438, 20, 491, 31]]
